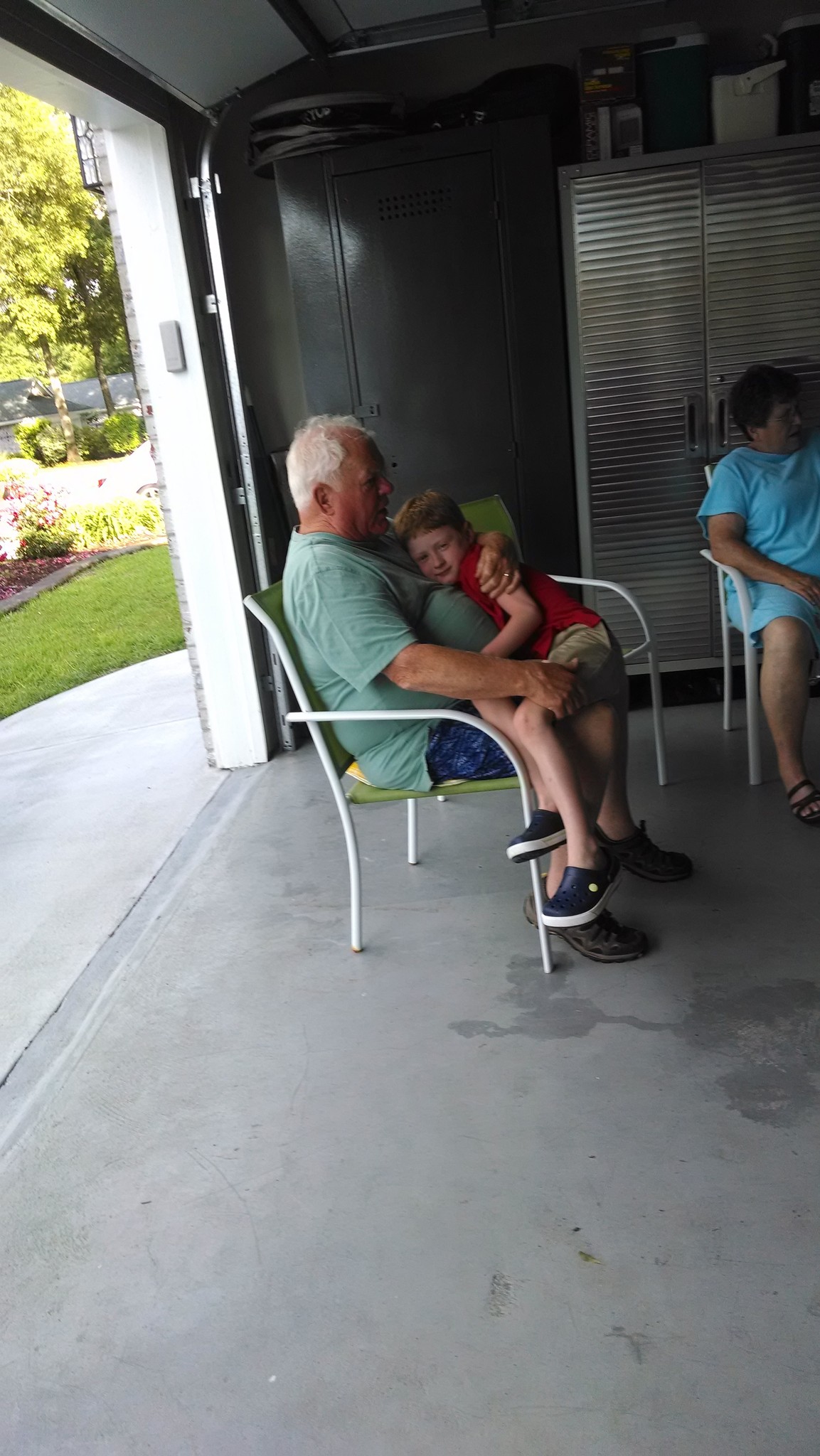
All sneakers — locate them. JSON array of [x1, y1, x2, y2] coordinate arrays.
[[524, 872, 648, 962], [588, 817, 694, 883]]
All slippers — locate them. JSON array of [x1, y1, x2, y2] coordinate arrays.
[[506, 809, 568, 864], [541, 846, 623, 928]]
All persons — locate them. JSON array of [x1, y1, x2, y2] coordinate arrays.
[[280, 415, 692, 964], [696, 362, 820, 820], [390, 489, 623, 926]]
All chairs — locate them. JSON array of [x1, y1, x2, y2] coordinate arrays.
[[243, 493, 666, 975], [698, 462, 820, 789]]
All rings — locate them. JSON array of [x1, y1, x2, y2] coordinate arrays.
[[504, 573, 512, 578]]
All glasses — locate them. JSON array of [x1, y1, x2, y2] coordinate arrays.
[[762, 399, 807, 424]]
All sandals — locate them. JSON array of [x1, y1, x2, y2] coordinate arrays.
[[786, 779, 820, 823]]
